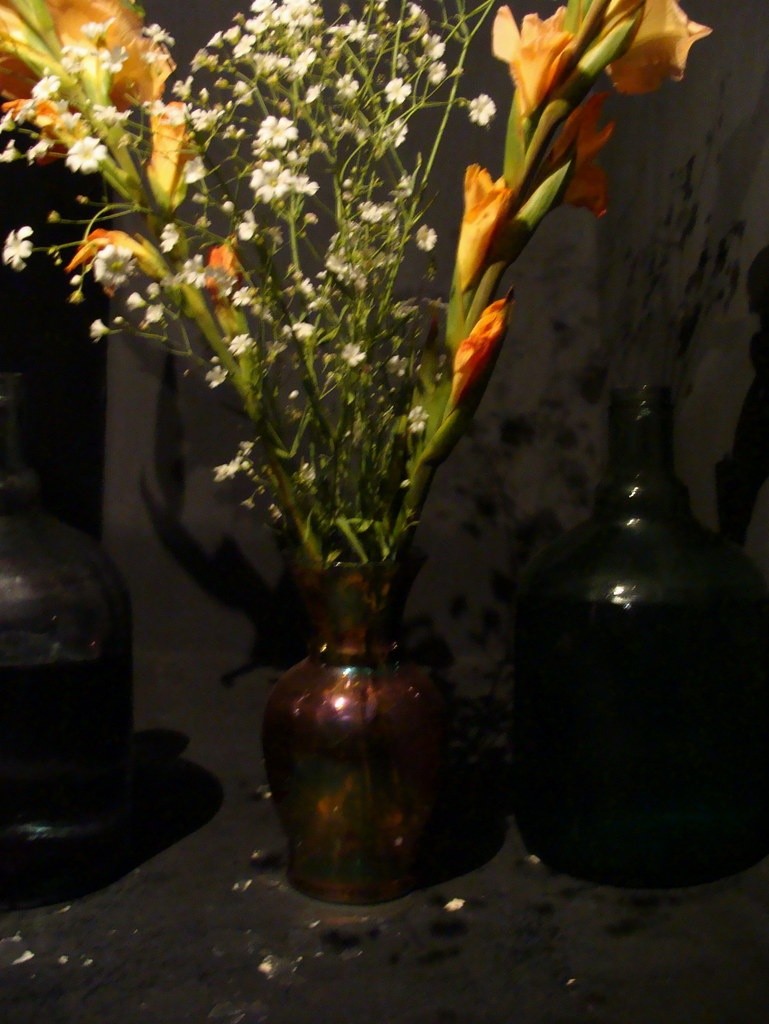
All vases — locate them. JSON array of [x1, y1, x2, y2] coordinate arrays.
[[0, 371, 135, 910], [261, 566, 436, 907], [515, 382, 746, 891]]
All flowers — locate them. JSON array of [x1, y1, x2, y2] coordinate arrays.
[[0, 0, 711, 567]]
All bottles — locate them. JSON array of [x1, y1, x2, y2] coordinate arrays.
[[513, 383, 769, 890], [0, 372, 135, 903]]
